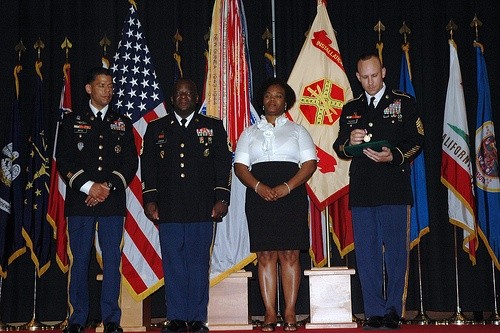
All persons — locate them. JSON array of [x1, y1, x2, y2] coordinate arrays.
[[332, 51, 426, 329], [234, 82, 318, 332], [54, 68, 139, 333], [139, 79, 232, 333]]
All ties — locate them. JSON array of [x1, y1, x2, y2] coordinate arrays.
[[368, 97, 375, 111], [96, 111, 102, 125], [180, 118, 187, 131]]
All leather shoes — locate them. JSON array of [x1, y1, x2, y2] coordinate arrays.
[[165, 320, 210, 333], [65, 324, 84, 333], [363, 312, 400, 332], [104, 322, 123, 333]]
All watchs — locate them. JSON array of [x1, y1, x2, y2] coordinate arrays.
[[105, 180, 114, 191]]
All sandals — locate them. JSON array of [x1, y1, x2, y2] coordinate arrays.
[[261, 310, 278, 333], [282, 312, 298, 332]]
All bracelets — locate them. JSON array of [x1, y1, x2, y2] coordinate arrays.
[[255, 181, 261, 194], [282, 182, 291, 194]]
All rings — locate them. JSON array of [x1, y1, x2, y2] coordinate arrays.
[[95, 200, 97, 201]]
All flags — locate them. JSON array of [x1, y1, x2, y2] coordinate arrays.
[[0, 57, 29, 281], [396, 42, 433, 250], [201, 0, 277, 285], [14, 59, 62, 276], [441, 37, 479, 269], [473, 38, 500, 284], [101, 0, 187, 303], [283, 1, 361, 269], [45, 60, 87, 274]]
